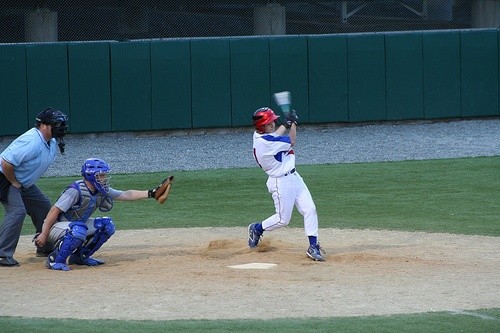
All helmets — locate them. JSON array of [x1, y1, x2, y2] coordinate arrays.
[[81, 158, 110, 193], [253, 107, 280, 134]]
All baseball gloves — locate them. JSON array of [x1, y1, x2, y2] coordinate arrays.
[[154, 175, 175, 205]]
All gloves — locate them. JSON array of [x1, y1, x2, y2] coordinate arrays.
[[290, 109, 298, 125], [283, 119, 291, 128]]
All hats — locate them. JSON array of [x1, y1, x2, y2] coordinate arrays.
[[36, 108, 53, 126]]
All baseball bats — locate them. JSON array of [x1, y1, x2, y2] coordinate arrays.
[[275, 91, 291, 126]]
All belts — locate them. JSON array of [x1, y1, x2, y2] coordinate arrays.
[[285, 169, 295, 176]]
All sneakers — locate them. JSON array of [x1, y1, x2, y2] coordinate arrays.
[[306, 244, 323, 261], [0, 257, 19, 265], [36, 248, 53, 257], [45, 261, 55, 268], [68, 258, 85, 265], [247, 223, 262, 248]]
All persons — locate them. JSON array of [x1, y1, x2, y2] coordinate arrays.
[[247, 107, 326, 262], [35, 157, 174, 271], [0, 106, 68, 266]]
